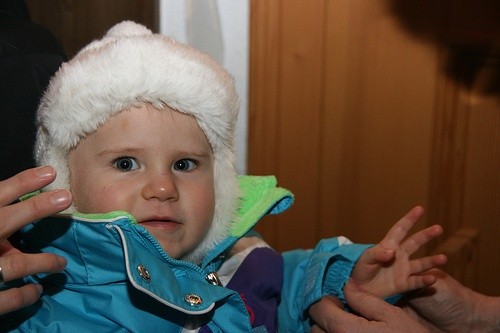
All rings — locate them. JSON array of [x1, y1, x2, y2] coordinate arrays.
[[0, 266, 5, 286]]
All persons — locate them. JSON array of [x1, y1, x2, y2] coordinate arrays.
[[308, 267, 500, 333], [0, 21, 448, 333], [0, 165, 72, 315]]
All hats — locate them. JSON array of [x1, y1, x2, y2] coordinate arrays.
[[34, 20, 241, 264]]
